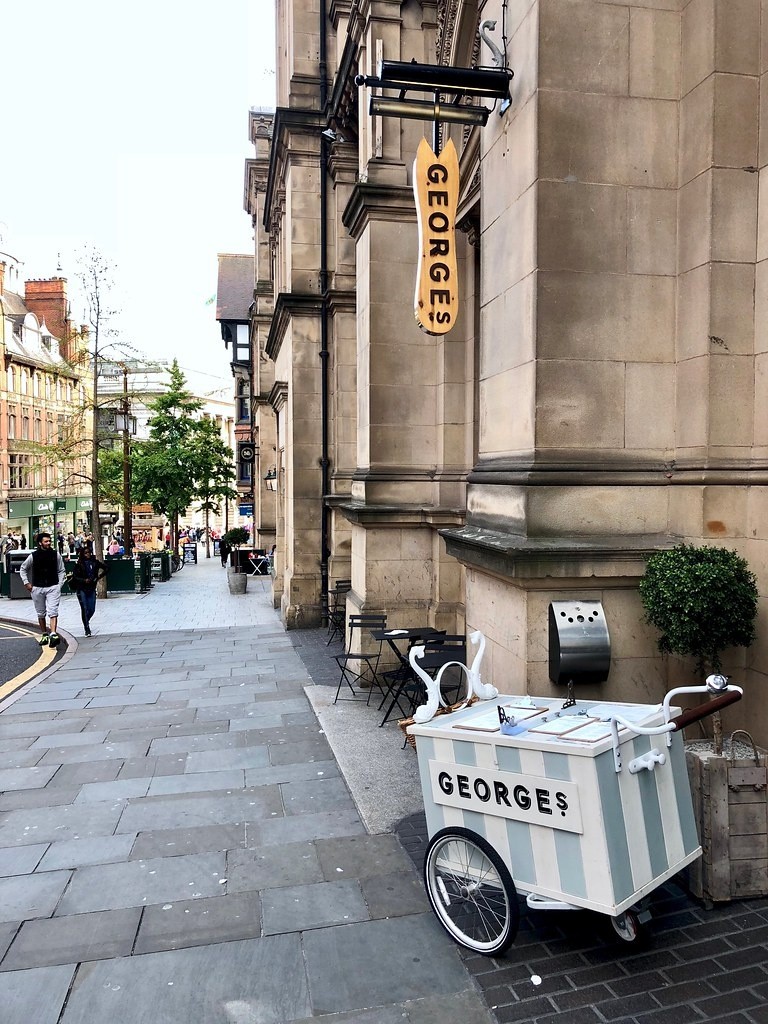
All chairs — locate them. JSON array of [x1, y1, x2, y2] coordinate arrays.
[[331, 615, 388, 706], [326, 580, 352, 635], [221, 527, 250, 595], [248, 545, 276, 576], [401, 633, 467, 750], [378, 629, 447, 729]]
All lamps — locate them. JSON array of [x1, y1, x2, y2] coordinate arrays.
[[263, 464, 276, 491]]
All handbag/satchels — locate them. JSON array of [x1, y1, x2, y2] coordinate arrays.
[[67, 574, 75, 593]]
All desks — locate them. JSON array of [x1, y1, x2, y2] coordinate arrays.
[[369, 627, 438, 727]]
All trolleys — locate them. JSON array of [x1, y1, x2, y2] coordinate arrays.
[[407, 629, 744, 955]]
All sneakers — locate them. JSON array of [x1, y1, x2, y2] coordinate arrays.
[[39, 634, 49, 645], [85, 628, 91, 637], [49, 633, 60, 647]]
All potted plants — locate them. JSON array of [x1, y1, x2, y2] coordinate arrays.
[[635, 541, 768, 910]]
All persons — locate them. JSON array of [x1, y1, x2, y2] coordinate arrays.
[[67, 531, 93, 555], [73, 547, 110, 638], [20, 534, 26, 550], [178, 525, 215, 547], [57, 531, 66, 554], [19, 533, 67, 648], [219, 536, 228, 568], [112, 532, 124, 546], [165, 532, 170, 548]]
